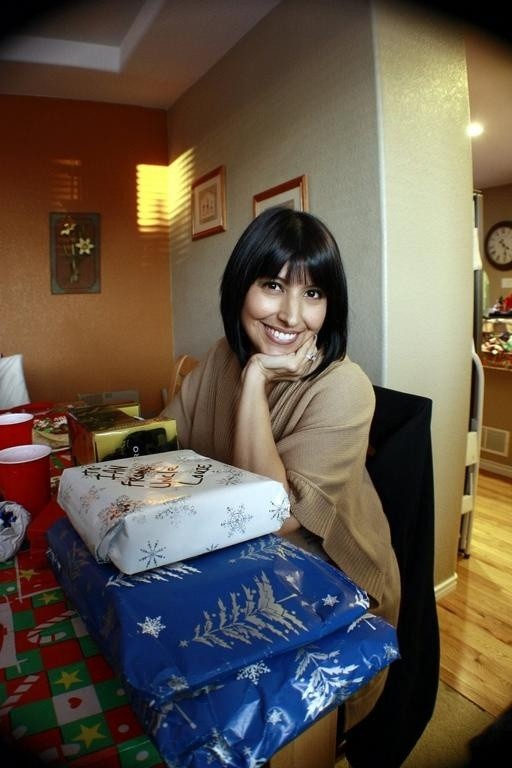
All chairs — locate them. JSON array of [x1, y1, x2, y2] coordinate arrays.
[[341, 384, 440, 768]]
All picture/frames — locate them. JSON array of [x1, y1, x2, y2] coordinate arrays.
[[48, 211, 101, 294], [191, 165, 226, 241], [253, 174, 309, 221]]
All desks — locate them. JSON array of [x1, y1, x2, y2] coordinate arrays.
[[0, 400, 340, 768]]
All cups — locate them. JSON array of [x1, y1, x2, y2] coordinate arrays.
[[0, 412, 54, 512]]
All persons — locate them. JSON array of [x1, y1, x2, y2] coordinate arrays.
[[152, 206, 403, 765]]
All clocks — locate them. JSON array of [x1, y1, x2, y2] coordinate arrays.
[[484, 220, 512, 271]]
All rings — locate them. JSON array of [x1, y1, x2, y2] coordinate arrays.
[[305, 354, 317, 362]]
